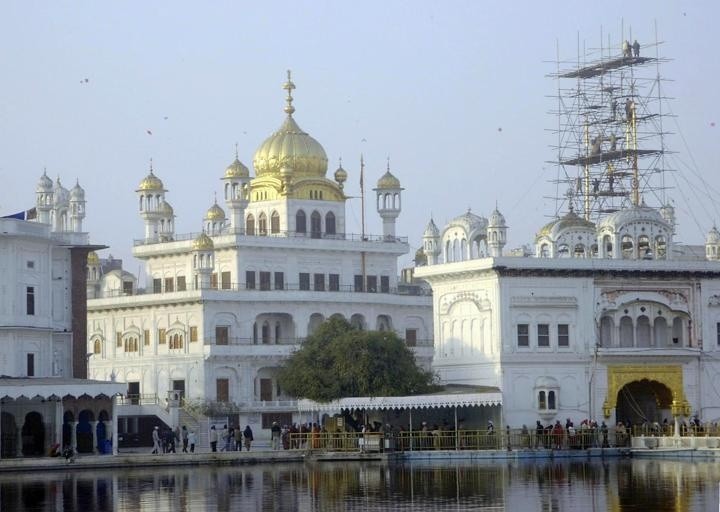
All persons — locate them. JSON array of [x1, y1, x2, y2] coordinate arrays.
[[518, 418, 720, 448], [270, 421, 282, 450], [243, 424, 254, 451], [208, 424, 218, 452], [633, 39, 640, 57], [591, 135, 604, 154], [218, 424, 230, 452], [610, 133, 621, 151], [234, 424, 243, 451], [575, 175, 583, 195], [623, 40, 629, 57], [607, 176, 614, 193], [484, 421, 495, 449], [611, 100, 619, 121], [592, 178, 601, 194], [627, 40, 634, 57], [150, 425, 162, 455], [159, 427, 168, 453], [620, 98, 633, 126], [282, 417, 467, 451], [70, 445, 78, 463], [167, 426, 182, 453], [228, 420, 236, 451], [62, 446, 74, 460], [187, 430, 197, 452], [181, 425, 189, 453]]
